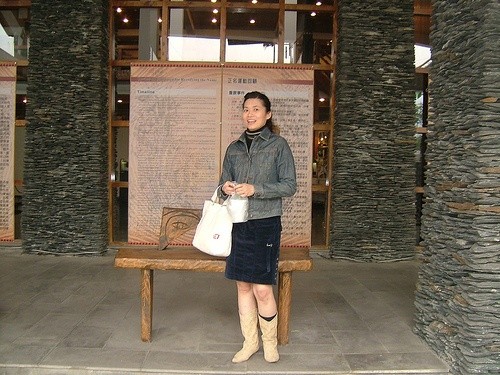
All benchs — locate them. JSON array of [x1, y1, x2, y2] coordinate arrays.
[[113, 207, 312, 345]]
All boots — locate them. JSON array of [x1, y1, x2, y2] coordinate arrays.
[[258, 311, 280, 363], [231, 309, 259, 363]]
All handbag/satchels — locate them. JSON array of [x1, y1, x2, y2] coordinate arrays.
[[191, 181, 241, 257], [227, 183, 248, 224]]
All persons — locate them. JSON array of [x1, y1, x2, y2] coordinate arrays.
[[216, 92, 296, 363]]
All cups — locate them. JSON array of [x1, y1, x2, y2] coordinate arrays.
[[230, 200, 247, 222]]
